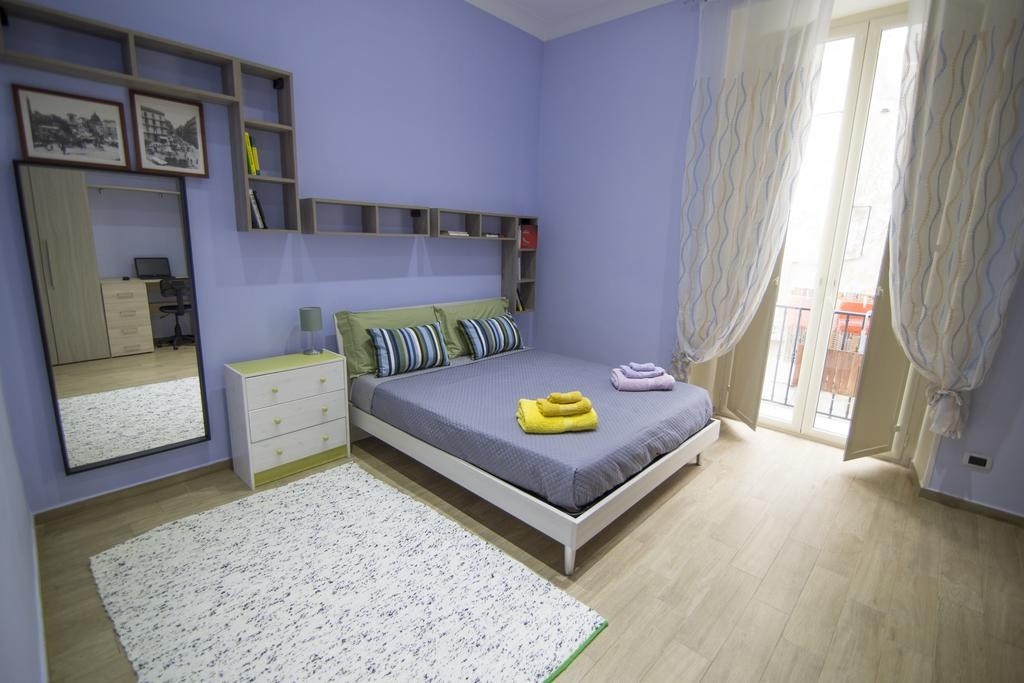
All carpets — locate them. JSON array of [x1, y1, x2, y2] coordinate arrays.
[[87, 463, 609, 683], [56, 376, 206, 468]]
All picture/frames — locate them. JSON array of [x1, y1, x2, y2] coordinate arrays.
[[127, 87, 209, 179], [11, 82, 131, 169]]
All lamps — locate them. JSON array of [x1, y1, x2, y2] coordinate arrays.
[[299, 307, 323, 356]]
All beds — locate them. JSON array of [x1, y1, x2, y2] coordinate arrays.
[[333, 296, 721, 575]]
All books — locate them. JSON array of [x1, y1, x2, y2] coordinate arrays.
[[245, 132, 261, 174], [440, 231, 470, 236], [518, 259, 522, 281], [250, 190, 267, 229], [485, 234, 501, 237], [516, 289, 524, 311]]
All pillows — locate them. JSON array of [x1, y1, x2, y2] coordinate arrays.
[[365, 321, 450, 378], [333, 306, 437, 379], [456, 312, 524, 360], [434, 298, 509, 360]]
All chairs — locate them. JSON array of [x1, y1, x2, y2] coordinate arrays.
[[155, 277, 195, 349]]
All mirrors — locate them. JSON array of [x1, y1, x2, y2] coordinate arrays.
[[12, 158, 212, 477]]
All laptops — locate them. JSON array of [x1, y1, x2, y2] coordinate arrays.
[[134, 257, 176, 279]]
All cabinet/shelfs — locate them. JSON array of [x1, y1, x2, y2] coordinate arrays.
[[100, 276, 188, 358], [224, 347, 351, 492], [300, 198, 539, 315], [0, 0, 302, 234], [20, 165, 110, 366]]
